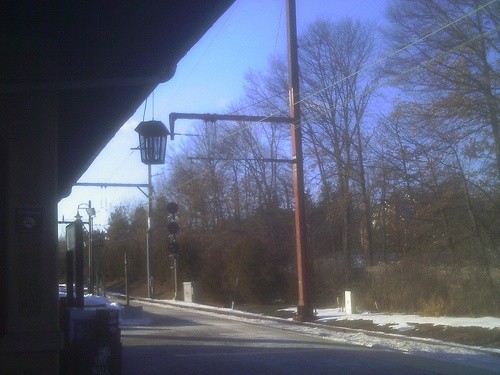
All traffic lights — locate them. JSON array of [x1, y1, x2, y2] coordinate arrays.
[[166, 201, 181, 255]]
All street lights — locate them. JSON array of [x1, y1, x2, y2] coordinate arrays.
[[75, 199, 95, 295]]
